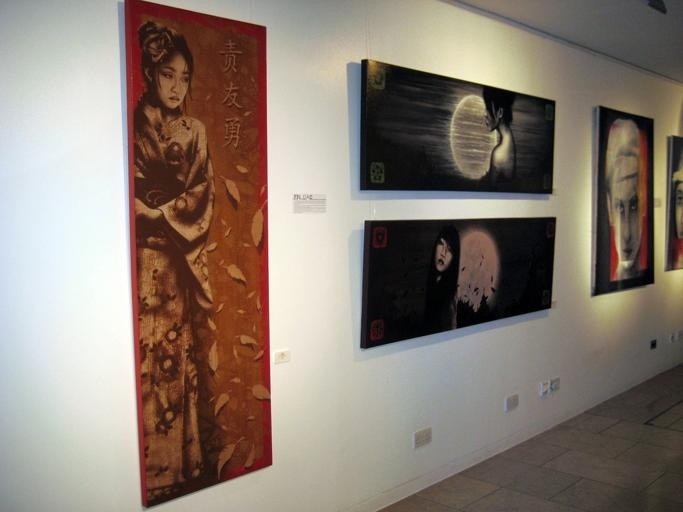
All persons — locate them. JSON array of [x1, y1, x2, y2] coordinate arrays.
[[604, 118, 648, 282], [395, 227, 461, 342], [483, 87, 516, 191], [673, 156, 683, 269], [132, 20, 223, 505]]
